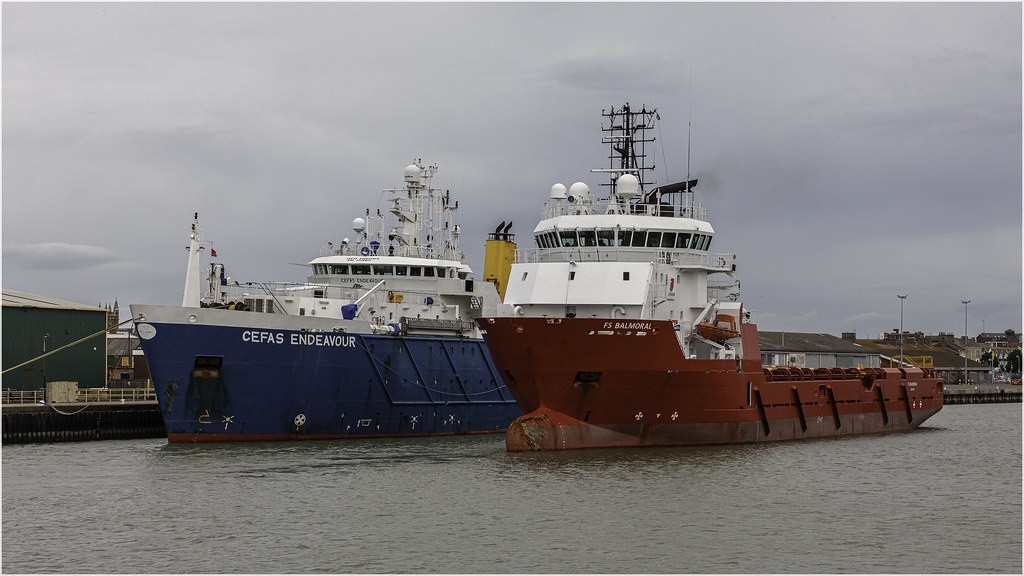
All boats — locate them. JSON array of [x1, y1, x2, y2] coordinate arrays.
[[694, 314, 740, 341], [127, 157, 520, 442], [474, 99, 943, 451]]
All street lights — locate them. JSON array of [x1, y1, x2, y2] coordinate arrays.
[[897, 294, 908, 366], [961, 300, 972, 384]]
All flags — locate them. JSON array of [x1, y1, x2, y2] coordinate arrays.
[[211, 248, 217, 257]]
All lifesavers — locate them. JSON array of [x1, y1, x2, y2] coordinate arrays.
[[717, 257, 725, 268], [669, 278, 674, 292]]
